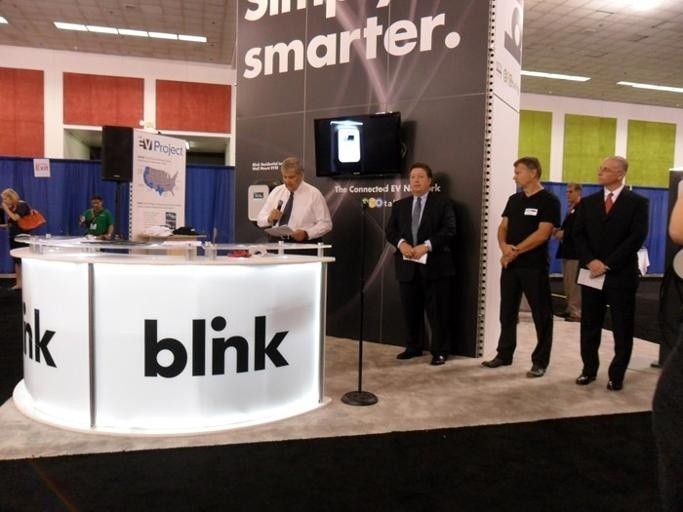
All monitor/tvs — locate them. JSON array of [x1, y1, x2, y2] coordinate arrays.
[[313, 111, 403, 177]]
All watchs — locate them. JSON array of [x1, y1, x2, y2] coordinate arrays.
[[423, 242, 430, 251]]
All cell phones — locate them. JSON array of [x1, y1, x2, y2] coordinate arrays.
[[329, 120, 364, 176]]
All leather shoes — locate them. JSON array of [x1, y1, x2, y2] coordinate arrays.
[[8, 287, 21, 292], [555, 312, 570, 318], [431, 355, 448, 365], [526, 365, 546, 377], [565, 316, 581, 322], [576, 374, 596, 385], [396, 348, 423, 360], [606, 380, 623, 391], [481, 355, 513, 368]]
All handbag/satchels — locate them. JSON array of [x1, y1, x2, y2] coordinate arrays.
[[16, 209, 47, 231]]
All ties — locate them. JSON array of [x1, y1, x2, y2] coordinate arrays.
[[412, 197, 422, 247], [605, 193, 614, 214], [279, 192, 294, 226]]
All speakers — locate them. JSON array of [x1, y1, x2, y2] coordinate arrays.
[[101, 125, 133, 183]]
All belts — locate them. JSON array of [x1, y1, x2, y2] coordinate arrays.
[[268, 234, 294, 240]]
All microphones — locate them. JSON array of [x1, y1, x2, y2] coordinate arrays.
[[272, 200, 283, 226]]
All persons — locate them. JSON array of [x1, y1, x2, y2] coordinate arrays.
[[564, 155, 650, 390], [481, 156, 560, 376], [651, 178, 683, 512], [79, 196, 115, 252], [385, 163, 457, 365], [552, 182, 583, 322], [257, 158, 333, 256], [0, 188, 30, 291]]
